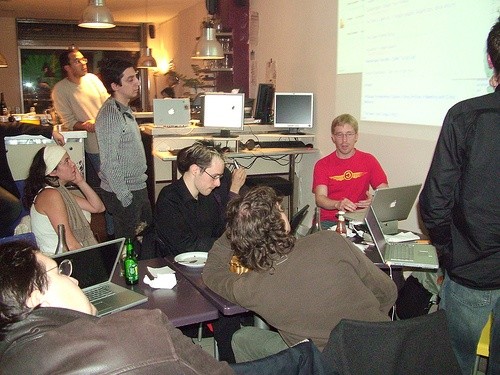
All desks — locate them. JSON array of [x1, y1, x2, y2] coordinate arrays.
[[165, 229, 431, 330], [111, 259, 219, 327], [145, 124, 320, 223]]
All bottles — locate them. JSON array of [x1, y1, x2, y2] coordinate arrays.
[[55, 225, 69, 255], [336, 211, 347, 238], [125, 237, 139, 286]]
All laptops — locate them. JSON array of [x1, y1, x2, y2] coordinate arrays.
[[337, 184, 422, 222], [365, 205, 439, 268], [52, 238, 148, 317], [153, 98, 192, 128], [289, 204, 310, 230]]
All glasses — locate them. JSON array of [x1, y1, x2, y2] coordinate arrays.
[[70, 59, 87, 66], [197, 164, 223, 181], [43, 259, 74, 277], [333, 133, 356, 138]]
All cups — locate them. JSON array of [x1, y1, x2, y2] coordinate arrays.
[[118, 242, 127, 276]]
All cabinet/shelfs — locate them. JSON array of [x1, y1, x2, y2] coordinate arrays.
[[199, 33, 233, 71]]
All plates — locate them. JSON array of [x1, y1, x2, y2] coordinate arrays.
[[174, 252, 208, 268]]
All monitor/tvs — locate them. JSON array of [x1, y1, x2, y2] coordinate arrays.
[[274, 92, 313, 135], [254, 83, 274, 124], [203, 93, 244, 137]]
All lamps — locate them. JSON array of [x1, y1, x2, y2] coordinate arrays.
[[191, 27, 225, 59], [78, 0, 116, 29], [136, 48, 157, 69], [0, 52, 8, 68]]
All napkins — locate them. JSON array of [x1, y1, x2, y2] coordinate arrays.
[[147, 265, 176, 277], [143, 274, 177, 289]]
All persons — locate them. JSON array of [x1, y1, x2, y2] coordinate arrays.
[[22, 143, 106, 258], [0, 240, 237, 375], [200, 185, 398, 361], [50, 48, 111, 245], [175, 147, 251, 211], [418, 17, 500, 375], [152, 139, 279, 364], [0, 122, 65, 202], [311, 115, 407, 323], [95, 57, 154, 261], [160, 88, 176, 99]]
